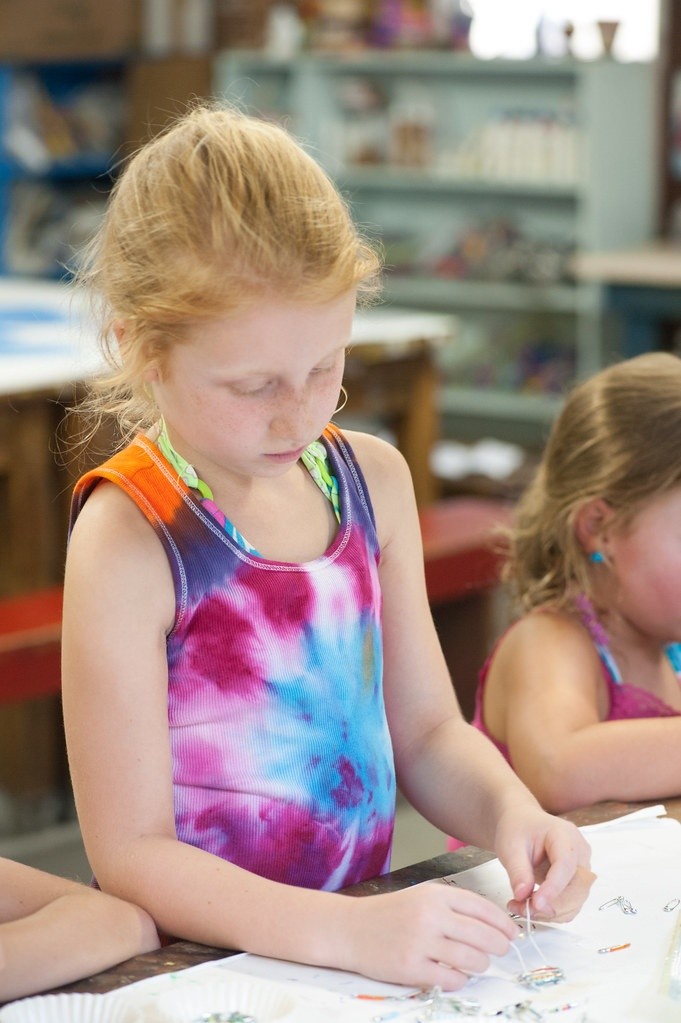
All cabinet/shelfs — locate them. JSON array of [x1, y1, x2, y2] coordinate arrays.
[[1, 44, 123, 282], [214, 46, 652, 432]]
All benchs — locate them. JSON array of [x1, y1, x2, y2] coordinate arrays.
[[3, 490, 519, 804]]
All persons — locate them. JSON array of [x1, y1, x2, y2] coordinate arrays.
[[58, 103, 596, 988], [471, 351, 680, 816], [1, 854, 161, 1005]]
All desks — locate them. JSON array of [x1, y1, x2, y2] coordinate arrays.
[[573, 239, 678, 370], [1, 797, 681, 1020], [3, 282, 438, 596]]
